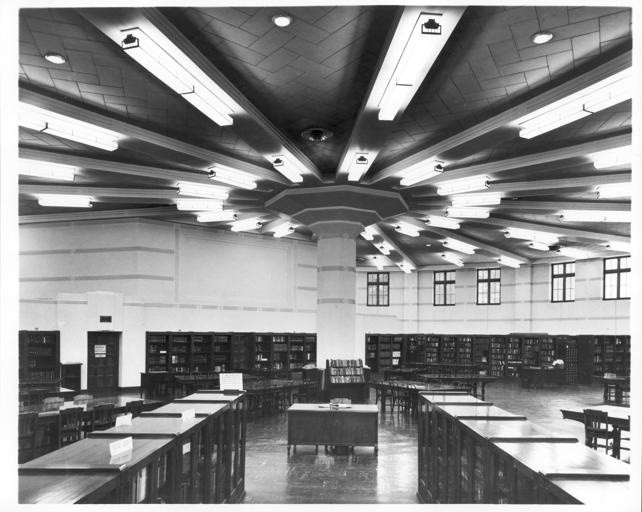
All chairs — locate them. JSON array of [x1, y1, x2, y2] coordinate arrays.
[[86, 403, 115, 432], [125, 400, 144, 418], [19, 412, 39, 464], [42, 396, 65, 404], [371, 364, 497, 414], [44, 407, 84, 449], [324, 397, 354, 452], [73, 394, 93, 401], [582, 408, 620, 459]]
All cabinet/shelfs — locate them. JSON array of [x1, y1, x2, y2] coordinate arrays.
[[324, 358, 370, 403], [61, 363, 83, 394], [365, 332, 631, 385], [416, 390, 629, 504], [19, 389, 247, 503], [19, 330, 61, 386], [145, 330, 318, 375]]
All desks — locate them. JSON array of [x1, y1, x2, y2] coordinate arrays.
[[560, 404, 630, 448], [20, 386, 77, 401], [288, 402, 379, 450], [516, 367, 564, 388], [371, 364, 497, 414], [20, 394, 164, 449], [590, 372, 629, 403], [171, 371, 320, 417]]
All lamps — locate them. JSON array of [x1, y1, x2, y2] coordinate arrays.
[[274, 224, 295, 238], [593, 145, 631, 171], [176, 182, 230, 200], [441, 253, 464, 266], [378, 12, 444, 121], [527, 242, 549, 252], [442, 238, 475, 255], [118, 27, 234, 127], [558, 210, 631, 223], [37, 196, 94, 208], [500, 227, 559, 245], [391, 220, 420, 238], [196, 209, 239, 223], [593, 182, 631, 199], [518, 68, 632, 140], [19, 156, 80, 182], [361, 223, 416, 275], [450, 193, 501, 206], [435, 176, 491, 196], [424, 215, 460, 230], [496, 257, 520, 269], [371, 256, 384, 272], [18, 101, 121, 152], [399, 160, 444, 187], [230, 217, 263, 232], [348, 153, 369, 182], [272, 155, 304, 183], [177, 200, 224, 211], [444, 208, 490, 219], [208, 166, 257, 190]]
